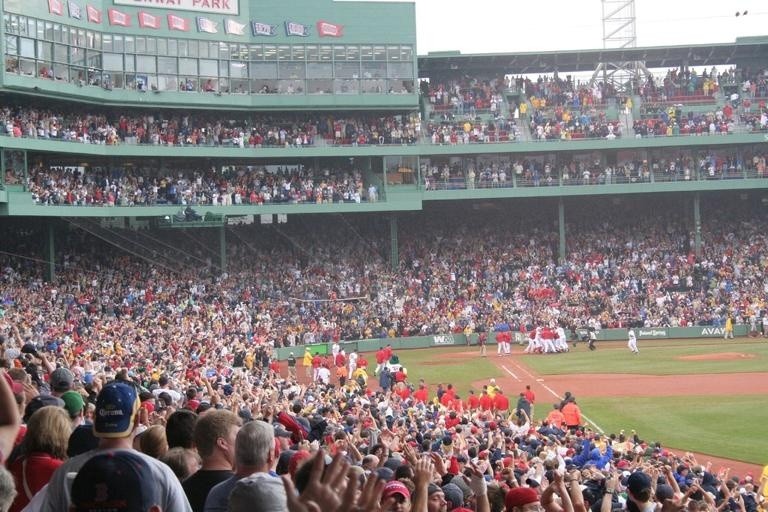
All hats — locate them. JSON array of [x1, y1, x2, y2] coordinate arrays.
[[381, 481, 410, 500], [591, 448, 600, 460], [616, 461, 626, 467], [621, 472, 652, 489], [410, 473, 472, 505], [187, 399, 200, 410], [92, 383, 141, 439], [68, 422, 98, 456], [22, 345, 36, 354], [142, 401, 156, 412], [504, 487, 538, 509], [274, 422, 293, 437], [656, 483, 673, 497], [72, 454, 155, 512], [24, 367, 85, 418], [478, 451, 488, 459], [278, 448, 309, 474]]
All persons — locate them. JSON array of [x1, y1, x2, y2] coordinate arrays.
[[1, 60, 768, 512]]
